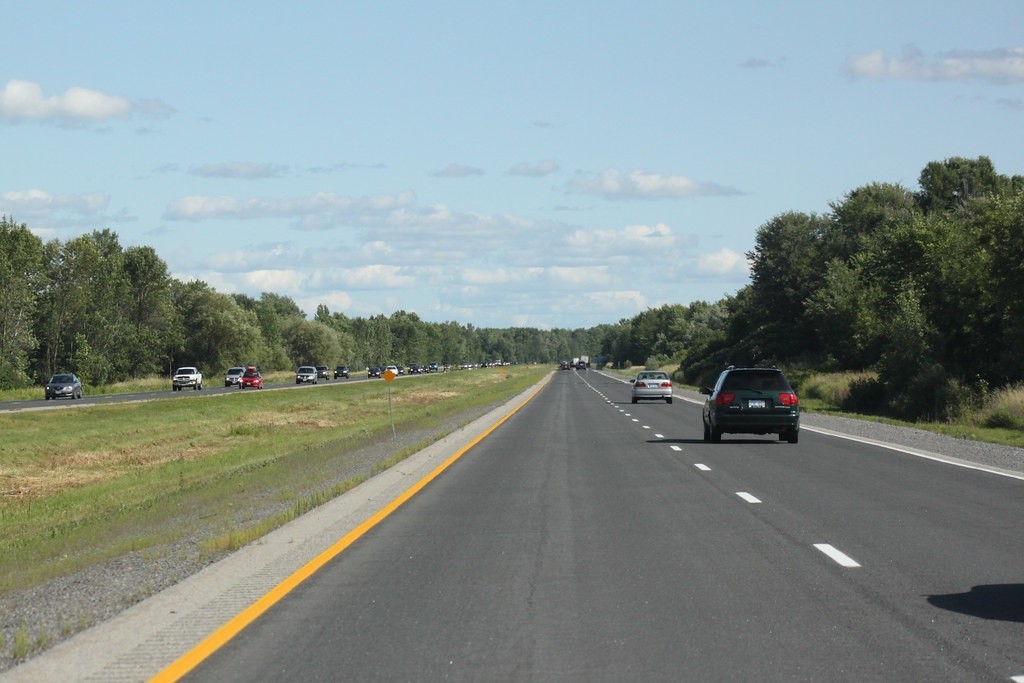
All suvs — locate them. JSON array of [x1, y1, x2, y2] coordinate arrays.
[[172, 366, 203, 391], [701, 364, 801, 444], [223, 367, 247, 387], [44, 372, 83, 400]]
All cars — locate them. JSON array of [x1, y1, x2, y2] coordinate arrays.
[[367, 367, 382, 379], [428, 362, 439, 372], [560, 355, 590, 371], [238, 366, 264, 389], [406, 363, 418, 375], [397, 366, 404, 375], [315, 366, 330, 380], [385, 365, 399, 376], [630, 370, 674, 404], [333, 364, 350, 379], [294, 366, 319, 384]]
